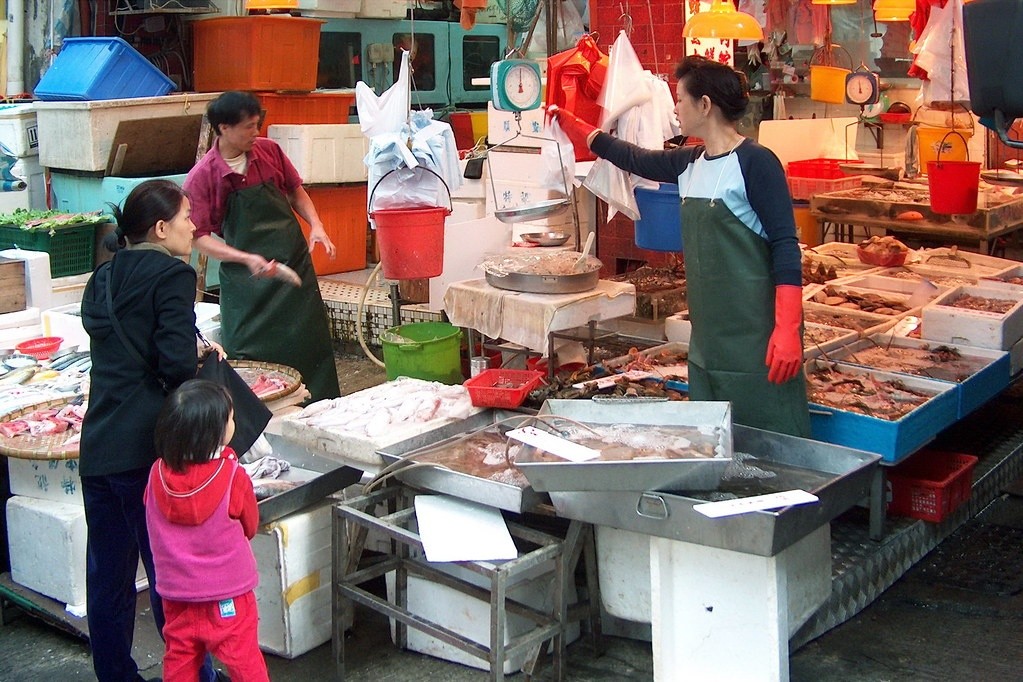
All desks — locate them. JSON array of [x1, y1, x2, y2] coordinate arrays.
[[459, 312, 600, 380]]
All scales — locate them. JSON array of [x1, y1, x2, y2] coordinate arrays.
[[483, 47, 574, 223], [857, 82, 892, 123], [837, 65, 889, 176]]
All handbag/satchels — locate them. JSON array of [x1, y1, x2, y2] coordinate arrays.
[[171, 348, 272, 461]]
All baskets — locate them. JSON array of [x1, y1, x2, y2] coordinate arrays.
[[463, 369, 545, 408], [787, 158, 865, 199], [888, 449, 978, 524], [857, 248, 907, 267], [16, 336, 63, 360]]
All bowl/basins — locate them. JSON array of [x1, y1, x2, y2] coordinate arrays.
[[879, 112, 911, 124]]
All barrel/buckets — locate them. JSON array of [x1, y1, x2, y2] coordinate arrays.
[[808, 44, 853, 104], [367, 165, 453, 280], [913, 103, 975, 174], [635, 182, 682, 252], [926, 131, 982, 214], [461, 344, 586, 386], [380, 321, 461, 386]]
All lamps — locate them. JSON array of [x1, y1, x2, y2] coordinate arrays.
[[812, 0, 858, 5], [682, 0, 764, 40], [872, 0, 916, 12], [874, 10, 914, 21]]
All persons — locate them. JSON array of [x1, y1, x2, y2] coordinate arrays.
[[548, 54, 813, 437], [184, 89, 342, 409], [81, 179, 228, 682], [144, 378, 274, 682]]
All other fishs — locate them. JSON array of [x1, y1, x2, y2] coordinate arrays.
[[250, 479, 306, 500], [0, 351, 92, 392], [296, 379, 466, 437]]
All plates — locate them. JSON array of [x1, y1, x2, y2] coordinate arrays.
[[520, 232, 572, 245], [886, 102, 911, 114]]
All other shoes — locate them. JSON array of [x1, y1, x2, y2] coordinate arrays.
[[147, 677, 162, 682], [214, 668, 231, 682]]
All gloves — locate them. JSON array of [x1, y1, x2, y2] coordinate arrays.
[[544, 106, 600, 150], [766, 284, 803, 384]]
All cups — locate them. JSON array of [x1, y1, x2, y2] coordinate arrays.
[[470, 357, 491, 378]]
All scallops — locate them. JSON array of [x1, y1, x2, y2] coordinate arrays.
[[812, 286, 913, 316]]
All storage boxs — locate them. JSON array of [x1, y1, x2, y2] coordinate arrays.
[[0, 0, 1023, 682]]
